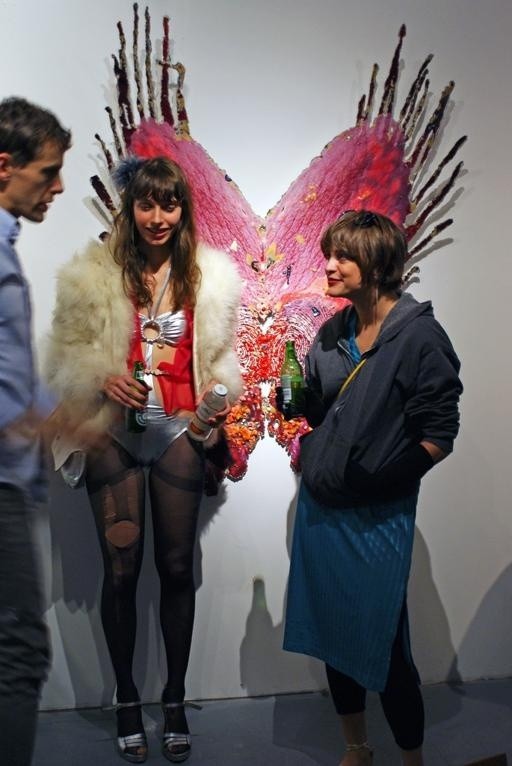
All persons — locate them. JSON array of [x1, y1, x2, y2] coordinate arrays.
[[276, 212, 462, 765], [42, 156, 245, 761], [2, 98, 73, 761]]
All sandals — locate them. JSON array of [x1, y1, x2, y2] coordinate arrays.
[[103, 686, 149, 763], [161, 690, 201, 760]]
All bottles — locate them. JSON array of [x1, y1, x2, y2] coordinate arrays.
[[186, 383, 227, 442], [126, 360, 149, 432], [281, 341, 306, 419]]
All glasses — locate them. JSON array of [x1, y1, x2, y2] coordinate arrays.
[[340, 212, 384, 229]]
